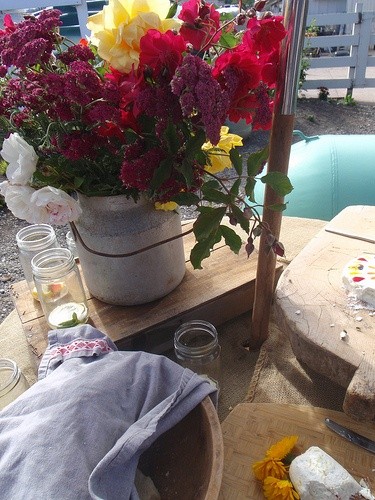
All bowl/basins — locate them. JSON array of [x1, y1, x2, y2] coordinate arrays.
[[137, 395, 224, 500]]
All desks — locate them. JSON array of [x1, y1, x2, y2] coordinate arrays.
[[1, 215, 375, 500]]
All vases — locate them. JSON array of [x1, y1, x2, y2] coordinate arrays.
[[71, 182, 188, 306]]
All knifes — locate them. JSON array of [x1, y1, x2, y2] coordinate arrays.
[[323, 417, 375, 452]]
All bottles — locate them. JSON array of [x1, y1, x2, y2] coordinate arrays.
[[15, 223, 68, 304], [173, 321, 221, 383], [0, 358, 30, 411], [31, 248, 90, 328]]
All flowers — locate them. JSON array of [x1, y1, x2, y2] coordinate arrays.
[[1, 0, 297, 268], [250, 434, 301, 500]]
